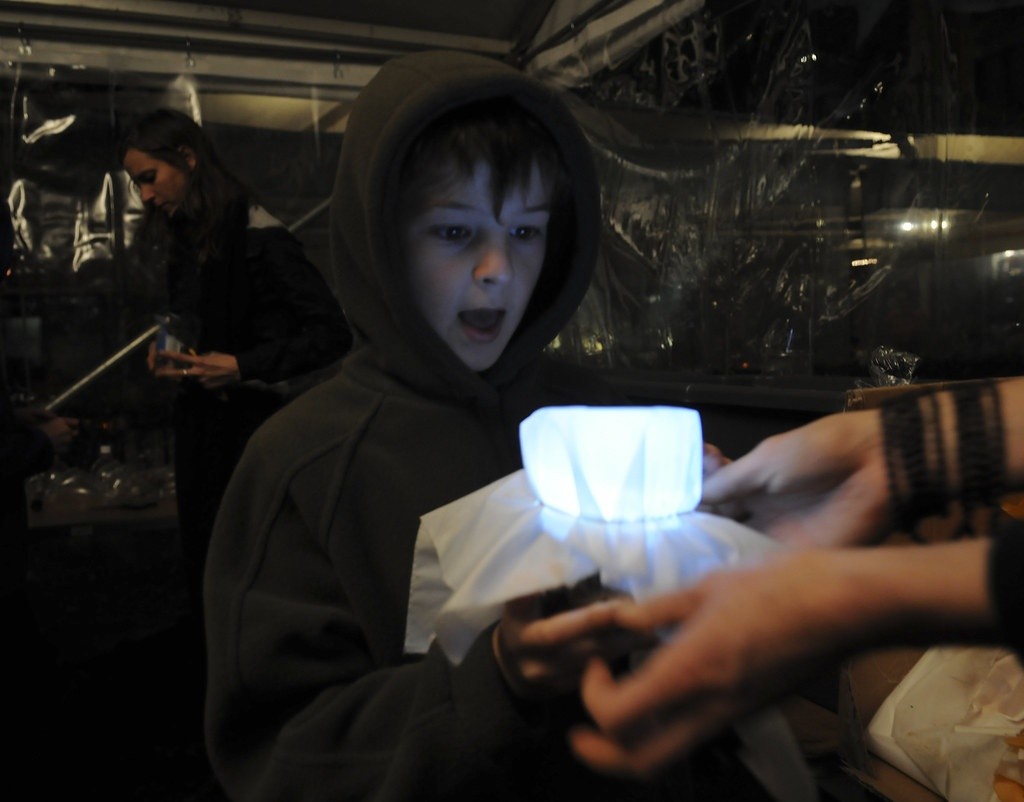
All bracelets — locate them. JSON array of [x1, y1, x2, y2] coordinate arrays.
[[881, 379, 1006, 523]]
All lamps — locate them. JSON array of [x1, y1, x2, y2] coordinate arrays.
[[332, 53, 344, 80], [15, 21, 32, 59], [184, 40, 195, 69], [872, 108, 940, 216]]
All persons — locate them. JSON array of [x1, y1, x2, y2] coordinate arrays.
[[118, 107, 354, 777], [566, 374, 1024, 784], [0, 192, 85, 777], [202, 52, 755, 801]]
